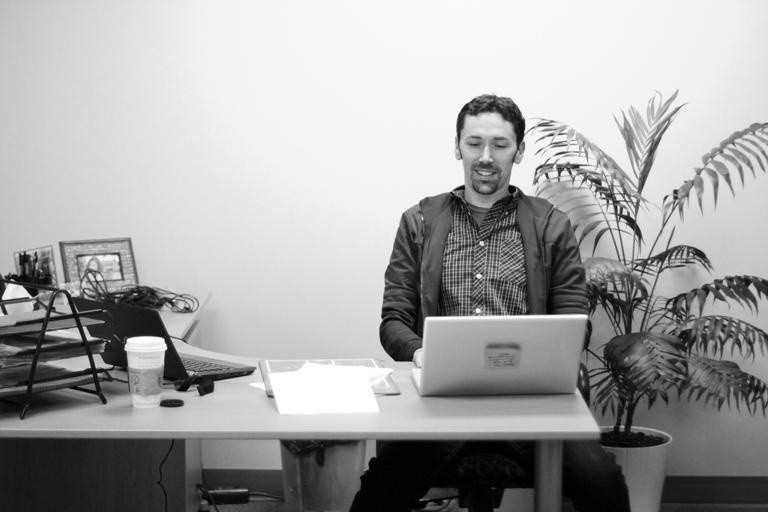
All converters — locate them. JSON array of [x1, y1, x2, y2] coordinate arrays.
[[202, 489, 249, 504]]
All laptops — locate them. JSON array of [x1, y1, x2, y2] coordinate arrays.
[[71, 297, 257, 385], [411, 314, 589, 396]]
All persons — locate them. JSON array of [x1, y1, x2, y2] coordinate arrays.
[[349, 91, 631, 512], [84, 254, 106, 279]]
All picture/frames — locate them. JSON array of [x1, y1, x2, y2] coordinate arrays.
[[59, 236, 141, 299]]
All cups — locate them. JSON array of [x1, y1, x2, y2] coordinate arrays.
[[122, 334, 168, 411]]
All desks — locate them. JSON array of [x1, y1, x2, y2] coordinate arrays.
[[0, 283, 603, 512]]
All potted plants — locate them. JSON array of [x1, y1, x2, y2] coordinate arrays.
[[519, 84, 768, 510]]
